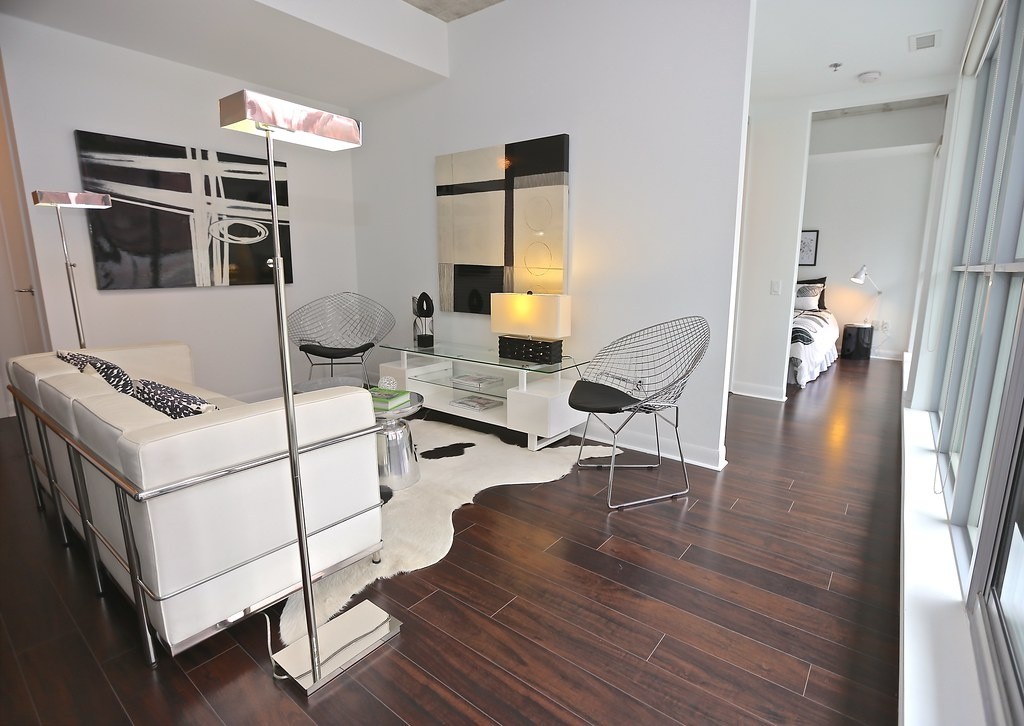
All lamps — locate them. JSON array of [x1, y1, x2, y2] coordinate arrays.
[[31, 189, 112, 210], [218, 88, 405, 696], [489, 291, 573, 366], [850, 264, 882, 294]]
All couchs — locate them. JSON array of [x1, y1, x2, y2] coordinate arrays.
[[5, 341, 384, 672]]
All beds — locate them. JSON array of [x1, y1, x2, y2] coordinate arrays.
[[787, 309, 840, 389]]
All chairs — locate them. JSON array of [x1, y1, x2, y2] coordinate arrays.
[[286, 292, 396, 389], [568, 316, 711, 510]]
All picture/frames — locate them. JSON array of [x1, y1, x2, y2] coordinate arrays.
[[798, 229, 819, 267]]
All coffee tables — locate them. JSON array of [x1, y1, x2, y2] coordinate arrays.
[[376, 335, 592, 454]]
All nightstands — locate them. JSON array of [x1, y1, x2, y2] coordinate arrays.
[[839, 323, 874, 361]]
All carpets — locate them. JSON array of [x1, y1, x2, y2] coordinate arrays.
[[278, 419, 625, 646]]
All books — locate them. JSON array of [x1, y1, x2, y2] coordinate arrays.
[[368, 387, 411, 411], [450, 372, 504, 389], [450, 395, 503, 413]]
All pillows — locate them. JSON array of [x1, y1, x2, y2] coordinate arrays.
[[794, 283, 825, 312], [797, 276, 827, 308], [57, 350, 134, 399], [129, 379, 220, 420]]
